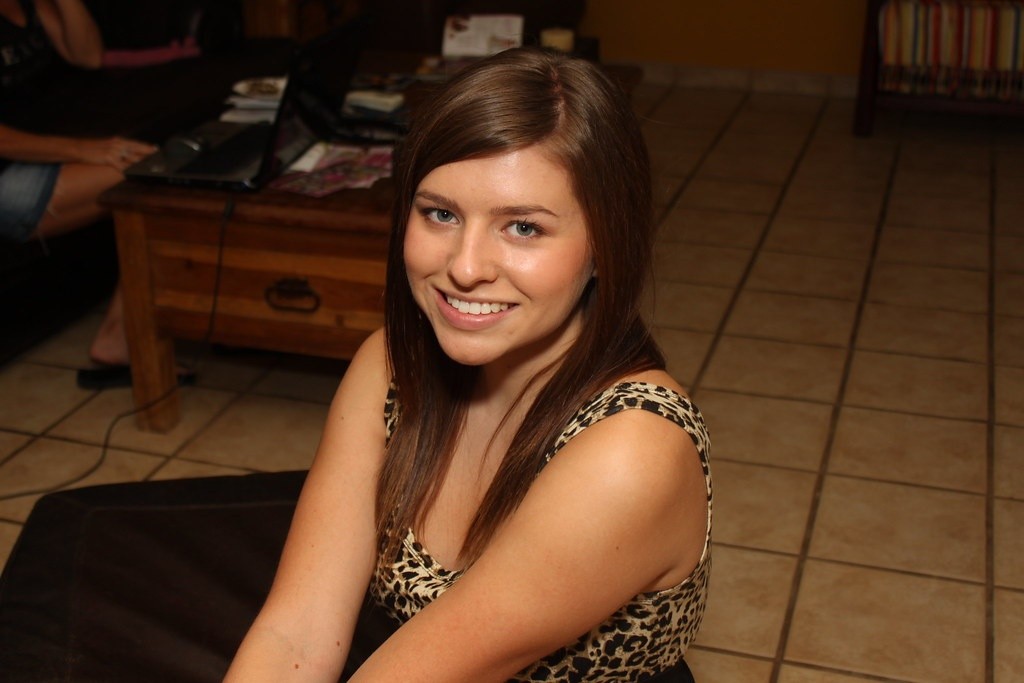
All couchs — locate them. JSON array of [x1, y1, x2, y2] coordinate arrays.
[[0, 32, 305, 365]]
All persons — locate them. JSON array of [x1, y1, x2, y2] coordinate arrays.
[[0, 48, 713, 683], [0, 0, 159, 385]]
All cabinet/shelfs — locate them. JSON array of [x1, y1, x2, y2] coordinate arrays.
[[241, 0, 363, 48]]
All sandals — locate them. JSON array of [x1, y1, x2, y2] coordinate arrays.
[[76, 349, 197, 388]]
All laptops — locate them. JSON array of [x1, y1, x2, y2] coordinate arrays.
[[120, 10, 372, 192]]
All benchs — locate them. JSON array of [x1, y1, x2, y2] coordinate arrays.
[[855, 0, 1024, 137], [0, 469, 311, 683]]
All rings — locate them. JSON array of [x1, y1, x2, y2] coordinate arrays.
[[121, 149, 129, 160]]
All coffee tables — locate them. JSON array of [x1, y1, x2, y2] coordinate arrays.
[[97, 51, 642, 432]]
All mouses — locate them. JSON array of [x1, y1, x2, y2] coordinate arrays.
[[168, 132, 208, 157]]
